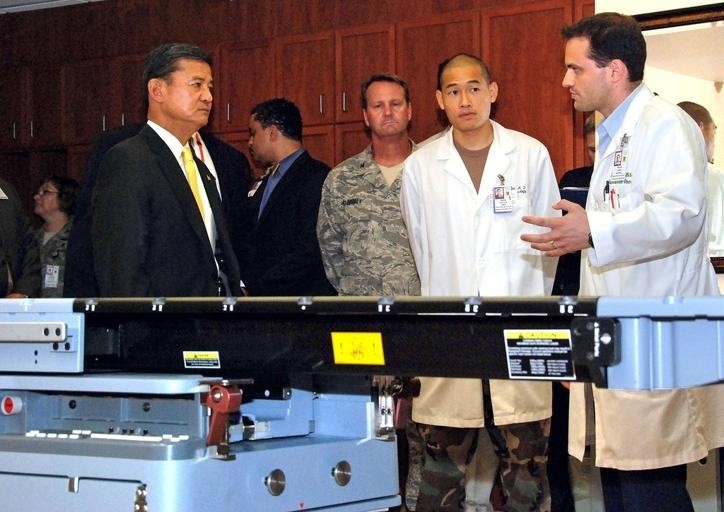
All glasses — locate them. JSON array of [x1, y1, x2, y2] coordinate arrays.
[[39, 189, 59, 196]]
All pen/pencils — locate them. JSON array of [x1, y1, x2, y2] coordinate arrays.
[[498, 175, 504, 185], [603, 181, 620, 209]]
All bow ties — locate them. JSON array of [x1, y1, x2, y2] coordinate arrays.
[[265, 162, 280, 176]]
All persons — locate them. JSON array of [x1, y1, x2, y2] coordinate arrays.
[[314, 72, 423, 296], [91, 42, 248, 296], [245, 97, 338, 296], [519, 11, 720, 295], [551, 113, 595, 296], [62, 119, 251, 296], [0, 177, 43, 298], [33, 175, 99, 298], [398, 52, 564, 296], [675, 101, 718, 165]]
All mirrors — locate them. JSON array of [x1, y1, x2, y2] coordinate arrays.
[[629, 2, 724, 273]]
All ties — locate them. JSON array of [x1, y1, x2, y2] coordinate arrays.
[[183, 147, 205, 222]]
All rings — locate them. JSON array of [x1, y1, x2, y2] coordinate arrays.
[[551, 240, 558, 249]]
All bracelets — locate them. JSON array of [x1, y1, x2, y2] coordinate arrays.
[[588, 232, 594, 248]]
